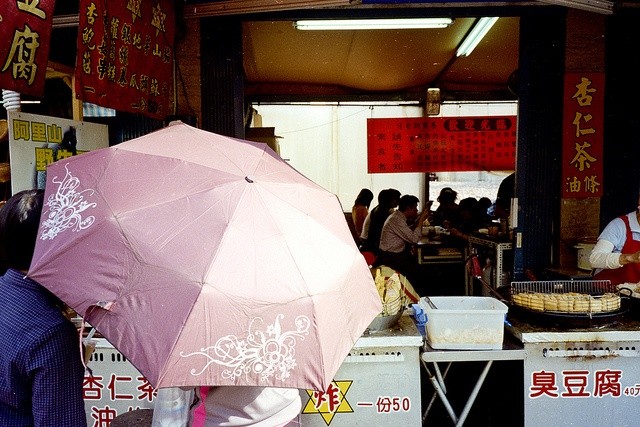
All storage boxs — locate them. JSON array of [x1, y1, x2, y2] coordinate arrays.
[[420, 294, 508, 349]]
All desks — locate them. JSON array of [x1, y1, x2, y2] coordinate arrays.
[[468, 236, 513, 296], [417, 241, 468, 296], [421, 343, 525, 426]]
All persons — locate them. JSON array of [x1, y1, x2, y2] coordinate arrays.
[[177, 387, 302, 427], [360, 189, 401, 243], [352, 189, 373, 243], [589, 204, 640, 293], [479, 197, 493, 214], [444, 198, 487, 240], [0, 190, 87, 427], [494, 173, 515, 219], [360, 252, 421, 308], [370, 190, 396, 256], [436, 188, 459, 216], [379, 195, 442, 297]]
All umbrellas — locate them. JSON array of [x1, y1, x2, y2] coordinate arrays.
[[23, 122, 384, 393]]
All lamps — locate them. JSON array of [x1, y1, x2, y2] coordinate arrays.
[[455, 17, 502, 57], [291, 19, 456, 31]]
